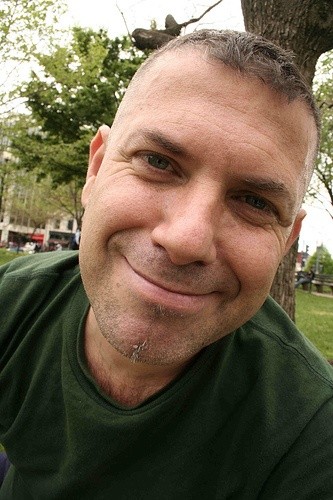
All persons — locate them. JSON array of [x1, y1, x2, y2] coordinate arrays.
[[0, 21, 333, 500]]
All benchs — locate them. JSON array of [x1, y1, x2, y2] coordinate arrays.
[[295, 271, 333, 294]]
